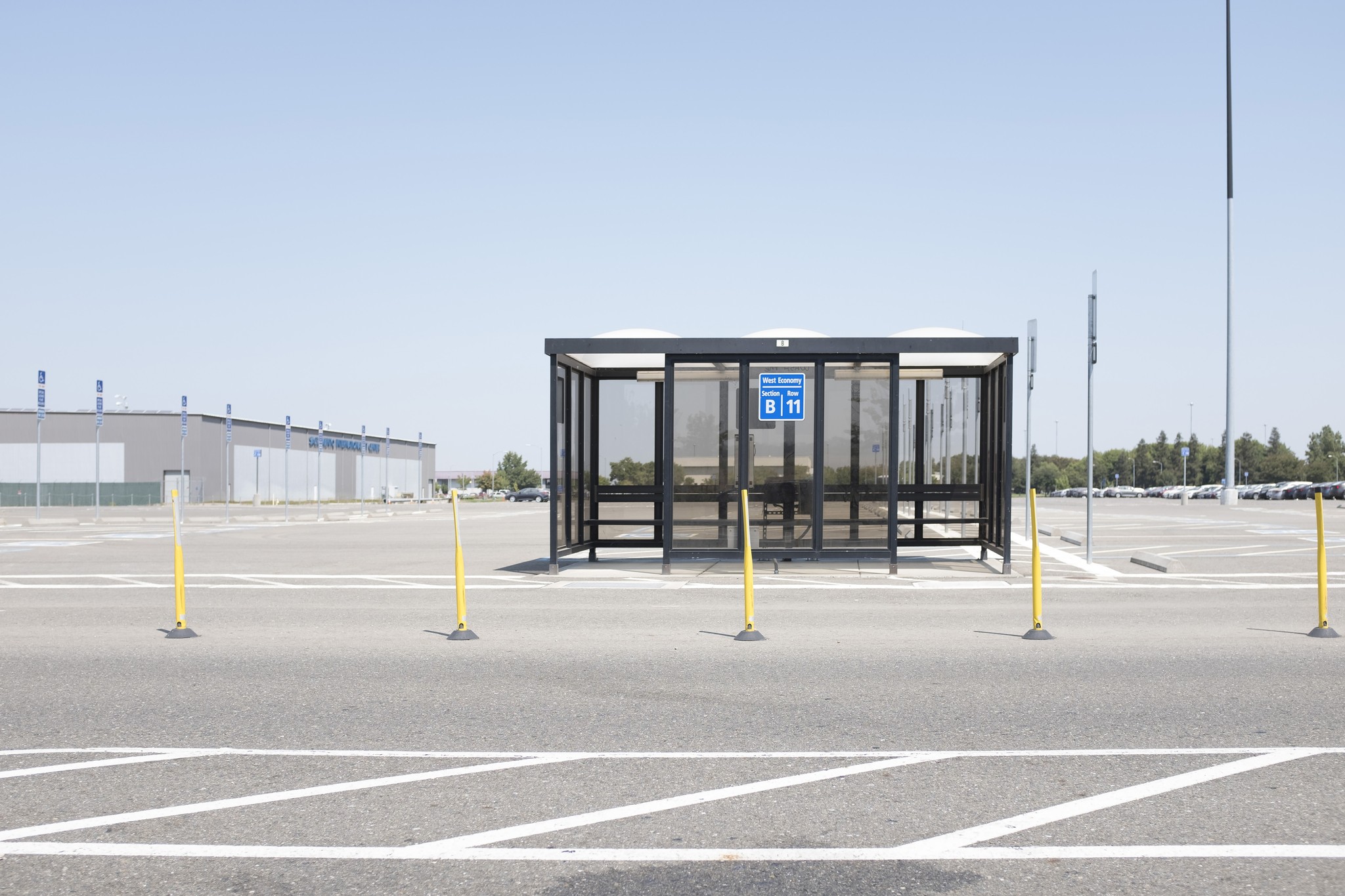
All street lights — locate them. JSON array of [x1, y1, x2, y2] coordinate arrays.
[[1054, 419, 1059, 456], [1153, 460, 1163, 473], [1264, 423, 1267, 443], [1189, 401, 1194, 436], [525, 444, 543, 488], [1127, 457, 1135, 488], [492, 451, 508, 496], [1328, 454, 1338, 481]]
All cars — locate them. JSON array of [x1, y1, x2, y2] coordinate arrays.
[[505, 487, 549, 502], [457, 488, 550, 499], [1050, 480, 1345, 502]]
